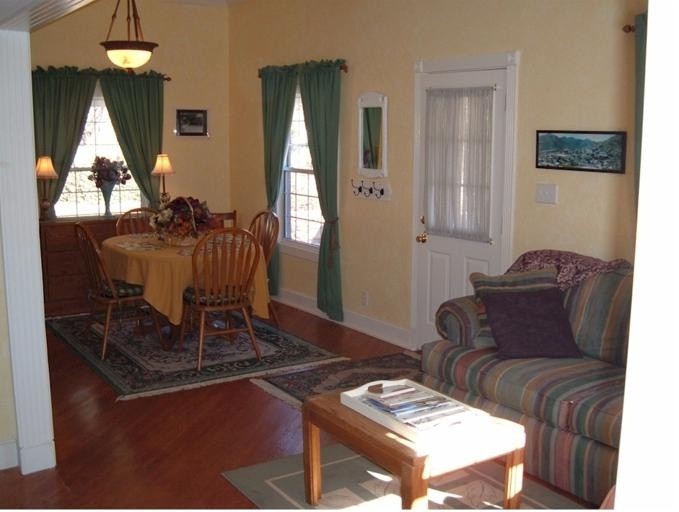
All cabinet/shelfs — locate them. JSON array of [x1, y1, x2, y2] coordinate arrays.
[[39, 213, 153, 317]]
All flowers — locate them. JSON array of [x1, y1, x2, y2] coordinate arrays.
[[149, 196, 223, 238], [88, 156, 131, 188]]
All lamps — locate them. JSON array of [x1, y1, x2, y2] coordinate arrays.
[[151, 154, 176, 203], [36, 156, 58, 221], [99, 0, 159, 69]]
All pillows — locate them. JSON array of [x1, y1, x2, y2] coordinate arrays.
[[470, 268, 584, 360]]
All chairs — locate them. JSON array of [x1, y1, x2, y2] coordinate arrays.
[[75, 207, 279, 372]]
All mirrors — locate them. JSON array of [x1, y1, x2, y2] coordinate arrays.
[[356, 91, 388, 179]]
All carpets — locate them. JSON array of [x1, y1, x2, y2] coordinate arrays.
[[250, 351, 424, 413], [221, 443, 589, 509], [46, 307, 352, 401]]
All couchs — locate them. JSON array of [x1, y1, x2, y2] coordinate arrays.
[[421, 249, 634, 506]]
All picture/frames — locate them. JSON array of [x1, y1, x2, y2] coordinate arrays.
[[177, 109, 207, 136], [535, 129, 626, 174]]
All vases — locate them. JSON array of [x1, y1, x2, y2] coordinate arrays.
[[100, 183, 115, 216]]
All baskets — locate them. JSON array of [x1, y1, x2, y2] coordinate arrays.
[[164, 196, 205, 246]]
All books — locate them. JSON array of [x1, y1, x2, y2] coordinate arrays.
[[354, 380, 472, 430]]
